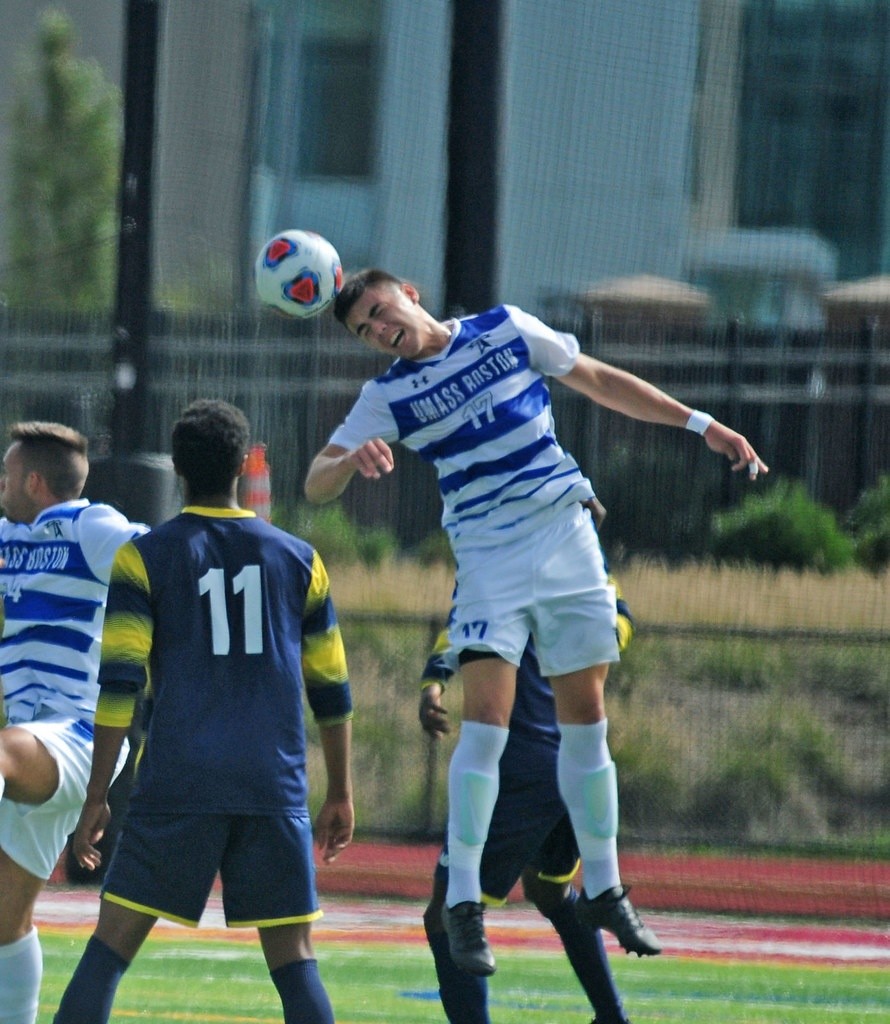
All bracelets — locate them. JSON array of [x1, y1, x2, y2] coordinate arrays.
[[685, 410, 715, 435]]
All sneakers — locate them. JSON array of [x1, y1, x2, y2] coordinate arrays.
[[440, 899, 495, 976], [573, 884, 661, 957]]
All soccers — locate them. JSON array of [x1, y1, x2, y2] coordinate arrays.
[[254, 228, 347, 320]]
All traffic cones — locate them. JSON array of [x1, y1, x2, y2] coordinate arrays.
[[244, 443, 275, 531]]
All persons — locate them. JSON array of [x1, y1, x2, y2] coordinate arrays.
[[0, 421, 152, 1023], [53, 398, 355, 1024], [306, 269, 768, 976], [418, 576, 635, 1023]]
